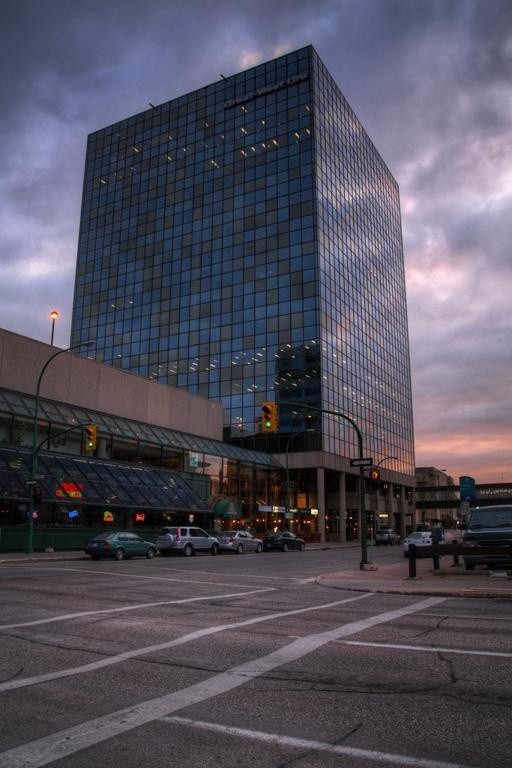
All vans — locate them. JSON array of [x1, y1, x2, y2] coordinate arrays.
[[460, 504, 512, 570]]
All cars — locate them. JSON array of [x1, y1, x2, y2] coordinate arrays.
[[403, 531, 447, 557], [375, 529, 401, 545], [217, 531, 305, 554], [85, 531, 157, 560]]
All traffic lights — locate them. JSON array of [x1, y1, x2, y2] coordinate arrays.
[[262, 401, 278, 432], [83, 425, 97, 452]]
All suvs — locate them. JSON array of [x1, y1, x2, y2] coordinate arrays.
[[155, 526, 219, 557]]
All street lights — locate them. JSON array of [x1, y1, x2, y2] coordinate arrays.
[[455, 520, 460, 528], [373, 457, 398, 539], [422, 470, 445, 530], [25, 340, 96, 554], [50, 311, 58, 345], [286, 427, 316, 513]]
